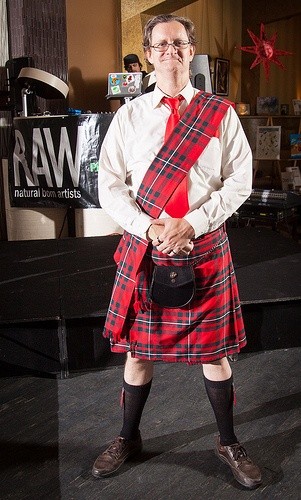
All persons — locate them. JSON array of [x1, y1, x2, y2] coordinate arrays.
[[120, 54, 155, 104], [91, 14, 263, 488]]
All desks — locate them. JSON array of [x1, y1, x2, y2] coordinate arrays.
[[0, 113, 128, 239], [238, 114, 301, 190]]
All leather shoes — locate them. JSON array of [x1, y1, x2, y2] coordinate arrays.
[[92, 431, 143, 478], [215, 435, 264, 488]]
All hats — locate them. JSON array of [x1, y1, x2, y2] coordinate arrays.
[[124, 54, 139, 65]]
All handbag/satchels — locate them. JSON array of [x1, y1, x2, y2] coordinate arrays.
[[148, 265, 195, 308]]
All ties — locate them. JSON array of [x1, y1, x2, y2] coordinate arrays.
[[161, 94, 190, 220]]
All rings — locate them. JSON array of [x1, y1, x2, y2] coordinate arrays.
[[173, 251, 176, 254], [157, 236, 161, 243]]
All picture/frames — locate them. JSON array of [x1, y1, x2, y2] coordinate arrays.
[[257, 126, 281, 160], [214, 58, 230, 96], [236, 102, 251, 116]]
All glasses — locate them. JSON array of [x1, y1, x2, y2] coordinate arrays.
[[150, 40, 190, 52]]
